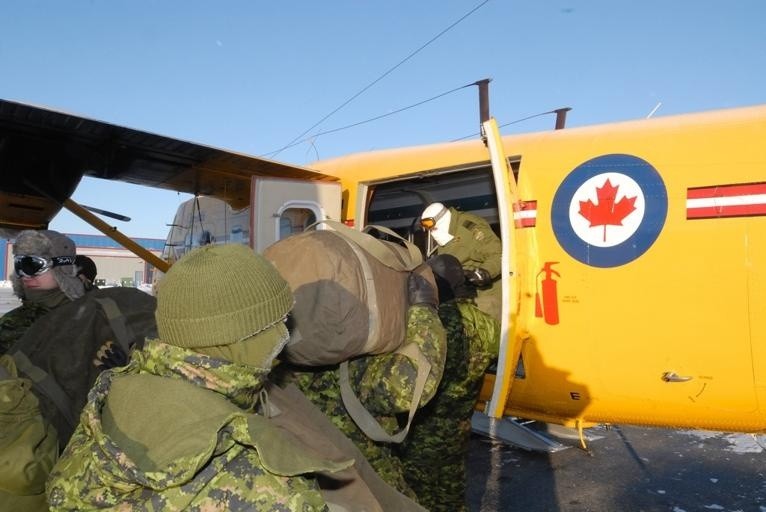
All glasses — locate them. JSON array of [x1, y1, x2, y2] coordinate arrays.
[[13, 254, 50, 278], [420, 217, 435, 229]]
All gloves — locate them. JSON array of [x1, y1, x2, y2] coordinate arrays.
[[408, 261, 440, 310], [465, 268, 493, 290], [93, 340, 137, 369]]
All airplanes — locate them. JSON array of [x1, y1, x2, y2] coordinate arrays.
[[1, 76, 766, 466]]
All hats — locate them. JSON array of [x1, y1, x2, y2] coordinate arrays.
[[153, 241, 296, 349], [426, 254, 478, 301], [420, 202, 455, 248], [10, 229, 85, 302]]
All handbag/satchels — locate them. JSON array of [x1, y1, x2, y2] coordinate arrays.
[[5, 286, 158, 455], [262, 219, 424, 367]]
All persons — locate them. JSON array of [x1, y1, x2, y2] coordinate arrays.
[[45, 242, 357, 512], [259, 201, 502, 512], [2, 229, 158, 512]]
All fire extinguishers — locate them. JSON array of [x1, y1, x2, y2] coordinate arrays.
[[535, 261, 561, 325]]
